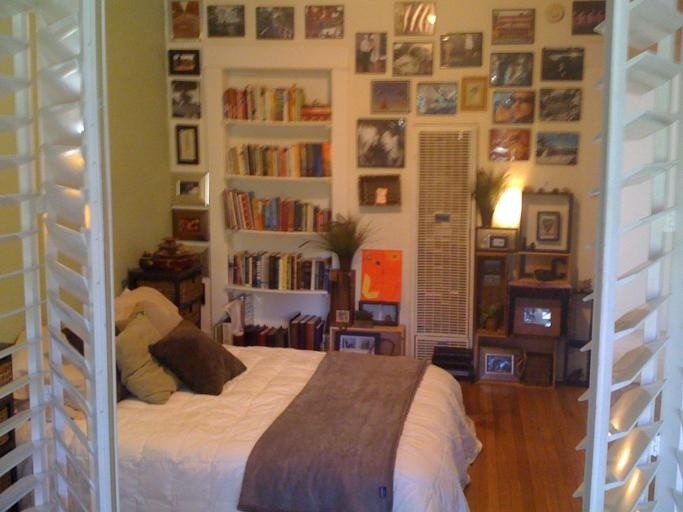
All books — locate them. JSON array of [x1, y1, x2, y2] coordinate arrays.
[[218, 73, 332, 355], [432, 341, 474, 382]]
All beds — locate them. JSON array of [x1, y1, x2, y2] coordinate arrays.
[[11, 339, 477, 511]]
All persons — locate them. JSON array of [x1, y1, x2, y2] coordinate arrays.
[[357, 33, 387, 63], [359, 121, 382, 166], [173, 0, 199, 39], [377, 119, 403, 166], [493, 91, 534, 123], [393, 46, 433, 75]]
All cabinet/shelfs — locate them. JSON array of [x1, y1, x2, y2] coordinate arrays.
[[473, 250, 574, 391], [219, 118, 334, 298]]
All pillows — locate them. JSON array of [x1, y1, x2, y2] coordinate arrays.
[[40, 283, 251, 422]]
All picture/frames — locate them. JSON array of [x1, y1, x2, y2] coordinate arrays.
[[335, 332, 380, 356], [354, 117, 407, 210], [357, 298, 402, 325], [480, 345, 525, 385], [535, 211, 560, 242], [367, 30, 489, 117], [511, 292, 569, 338], [169, 50, 203, 167]]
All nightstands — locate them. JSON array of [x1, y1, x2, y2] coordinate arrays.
[[130, 264, 206, 331]]
